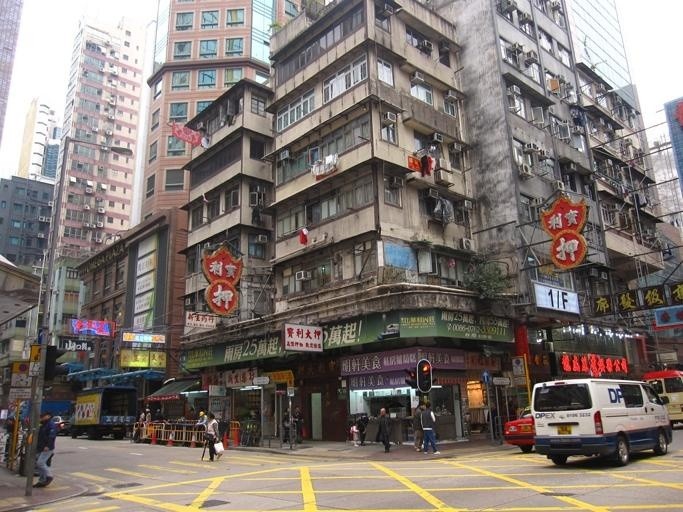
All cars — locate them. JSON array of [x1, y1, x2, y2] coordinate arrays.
[[504, 407, 536, 453], [52, 416, 70, 435]]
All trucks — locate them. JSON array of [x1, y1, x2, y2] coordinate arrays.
[[69, 385, 138, 440]]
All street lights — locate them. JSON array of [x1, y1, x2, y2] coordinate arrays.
[[26, 136, 132, 498]]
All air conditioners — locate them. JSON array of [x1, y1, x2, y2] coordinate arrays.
[[379, 2, 475, 255], [38, 201, 54, 255], [196, 121, 310, 281], [68, 39, 122, 244], [500, 1, 574, 194], [598, 271, 608, 283]]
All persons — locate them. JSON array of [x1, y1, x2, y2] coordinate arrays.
[[33, 409, 57, 488], [206, 411, 223, 461], [190, 406, 198, 420], [412, 400, 440, 455], [283, 408, 291, 444], [292, 407, 305, 441], [153, 408, 164, 443], [197, 411, 209, 427], [3, 415, 17, 461], [373, 408, 392, 452], [139, 408, 145, 424], [145, 408, 152, 422]]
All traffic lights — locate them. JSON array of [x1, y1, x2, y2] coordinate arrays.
[[405, 359, 436, 394]]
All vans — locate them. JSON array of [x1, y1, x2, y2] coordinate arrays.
[[643, 371, 683, 429], [531, 379, 672, 468]]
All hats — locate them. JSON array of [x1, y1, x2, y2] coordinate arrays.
[[40, 410, 52, 416]]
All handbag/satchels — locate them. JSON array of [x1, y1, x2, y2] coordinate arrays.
[[213, 441, 225, 455]]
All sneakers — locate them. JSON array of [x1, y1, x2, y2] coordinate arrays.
[[33, 477, 52, 487], [416, 449, 440, 454]]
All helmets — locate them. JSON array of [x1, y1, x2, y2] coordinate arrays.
[[199, 412, 204, 417]]
